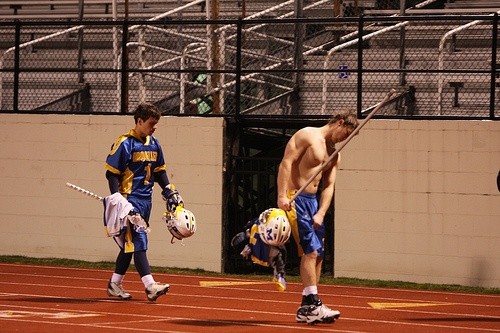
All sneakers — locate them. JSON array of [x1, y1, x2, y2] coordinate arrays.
[[106, 278, 132, 299], [144, 281, 170, 302], [296, 294, 336, 323], [306, 295, 340, 325]]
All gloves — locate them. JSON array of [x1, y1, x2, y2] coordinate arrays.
[[160, 184, 184, 212]]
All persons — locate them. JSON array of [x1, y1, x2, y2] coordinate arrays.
[[277, 114, 360, 326], [105, 102, 184, 302]]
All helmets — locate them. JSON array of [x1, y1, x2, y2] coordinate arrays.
[[260, 209, 291, 247], [165, 206, 196, 240]]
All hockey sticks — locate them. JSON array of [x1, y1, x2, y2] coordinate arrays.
[[66, 182, 105, 203], [281, 88, 396, 209]]
